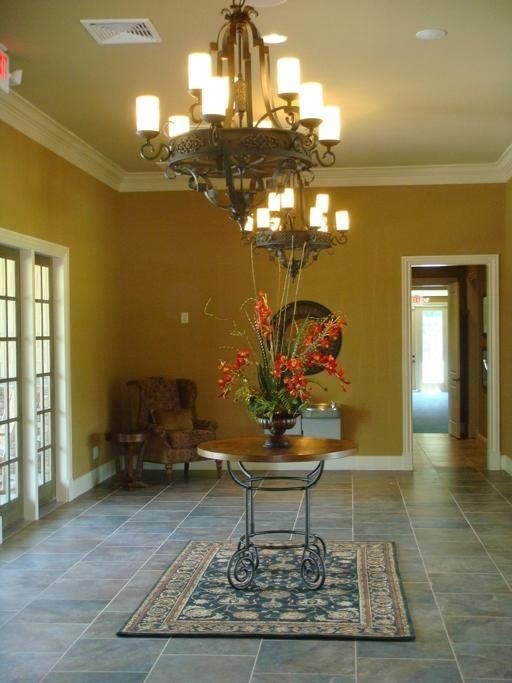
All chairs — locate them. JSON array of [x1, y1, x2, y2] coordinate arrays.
[[128, 376, 222, 484]]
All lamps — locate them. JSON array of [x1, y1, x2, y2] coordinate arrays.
[[239, 162, 349, 282], [136, 3, 347, 227]]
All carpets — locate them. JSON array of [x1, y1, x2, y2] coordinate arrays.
[[116, 540, 416, 642]]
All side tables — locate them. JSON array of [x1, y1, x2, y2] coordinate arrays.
[[106, 432, 149, 491]]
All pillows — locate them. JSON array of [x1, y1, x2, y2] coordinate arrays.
[[160, 408, 194, 430]]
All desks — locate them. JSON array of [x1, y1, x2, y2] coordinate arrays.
[[197, 438, 358, 590]]
